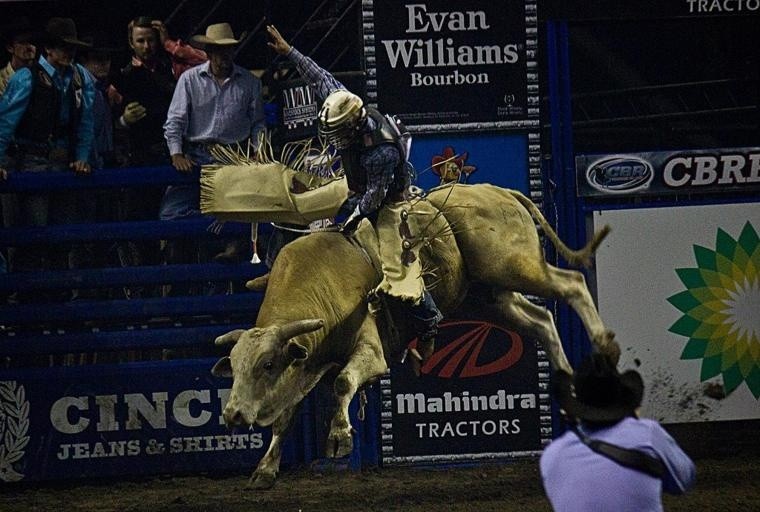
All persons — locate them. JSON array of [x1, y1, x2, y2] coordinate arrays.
[[162, 22, 270, 328], [539, 356, 696, 511], [256, 71, 286, 153], [0, 16, 208, 367], [200, 21, 443, 364]]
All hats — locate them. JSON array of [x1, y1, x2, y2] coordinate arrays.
[[192, 22, 240, 46], [33, 16, 93, 49], [554, 351, 645, 424]]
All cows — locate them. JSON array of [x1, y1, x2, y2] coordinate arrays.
[[211, 183, 621, 491]]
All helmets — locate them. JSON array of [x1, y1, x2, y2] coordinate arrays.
[[316, 88, 368, 150]]
